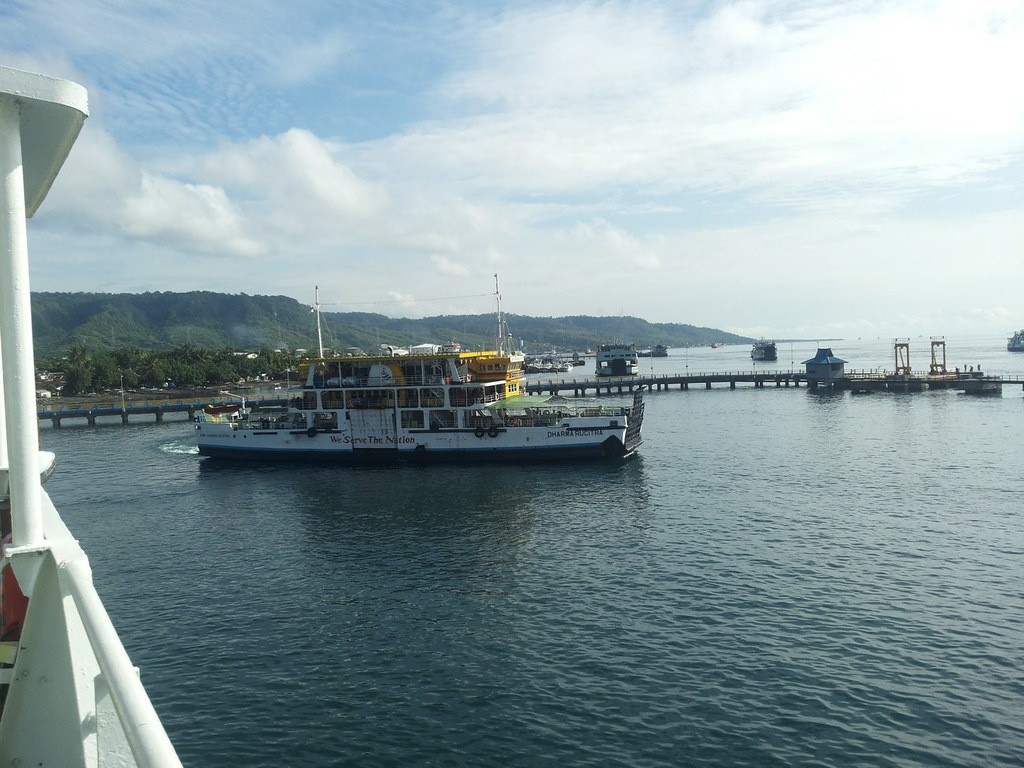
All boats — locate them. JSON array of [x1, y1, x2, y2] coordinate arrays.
[[191, 273, 646, 456], [750, 337, 778, 360], [525, 352, 585, 373], [1006, 328, 1024, 351], [712, 342, 720, 349], [637, 345, 669, 357], [585, 346, 592, 354], [595, 338, 639, 374]]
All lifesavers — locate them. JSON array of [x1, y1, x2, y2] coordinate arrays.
[[466, 374, 471, 382], [488, 428, 498, 438], [445, 375, 451, 384], [415, 445, 425, 455], [475, 428, 487, 438], [308, 428, 316, 437], [495, 394, 499, 400]]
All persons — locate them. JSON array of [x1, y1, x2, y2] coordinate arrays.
[[261, 414, 305, 429], [507, 408, 562, 427]]
